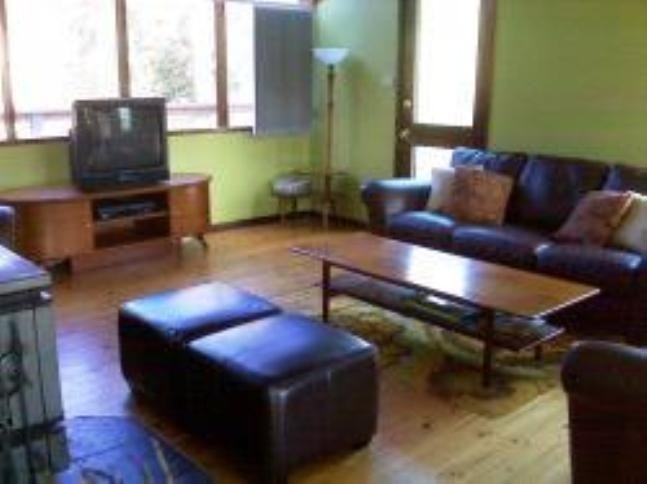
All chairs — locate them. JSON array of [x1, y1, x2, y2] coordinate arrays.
[[561, 339, 647, 484]]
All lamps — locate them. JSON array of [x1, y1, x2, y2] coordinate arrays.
[[309, 48, 348, 230]]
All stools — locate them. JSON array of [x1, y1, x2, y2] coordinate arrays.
[[182, 312, 378, 484], [272, 172, 355, 229], [119, 280, 282, 433]]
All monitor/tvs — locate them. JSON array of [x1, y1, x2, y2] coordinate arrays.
[[70, 97, 170, 186]]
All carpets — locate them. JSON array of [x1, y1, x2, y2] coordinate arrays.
[[269, 280, 625, 418], [48, 415, 217, 483]]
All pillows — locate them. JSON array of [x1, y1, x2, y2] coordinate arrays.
[[551, 190, 647, 256], [425, 167, 514, 227]]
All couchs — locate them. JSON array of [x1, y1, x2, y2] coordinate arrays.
[[361, 146, 646, 350]]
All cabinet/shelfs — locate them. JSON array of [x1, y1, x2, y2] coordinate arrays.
[[1, 246, 70, 484], [1, 175, 210, 288]]
